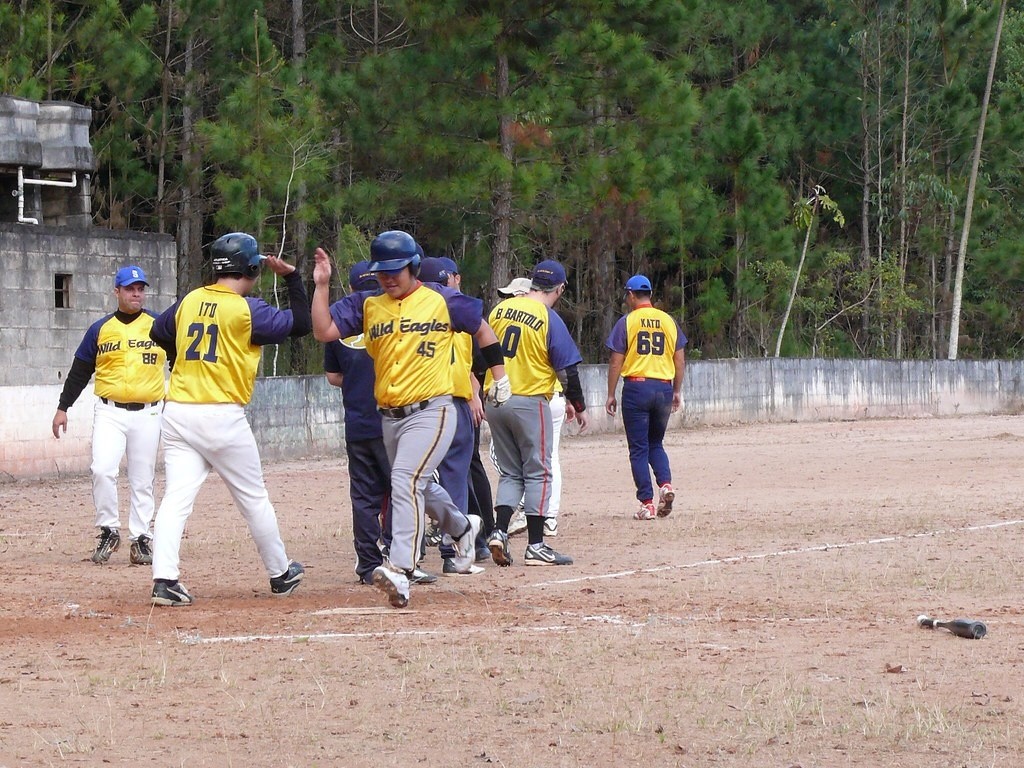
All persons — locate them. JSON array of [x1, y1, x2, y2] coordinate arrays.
[[311, 230, 511, 608], [53, 266, 168, 565], [324, 242, 588, 586], [149, 232, 311, 607], [604, 274, 688, 519]]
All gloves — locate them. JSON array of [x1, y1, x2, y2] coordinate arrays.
[[488, 375, 512, 408]]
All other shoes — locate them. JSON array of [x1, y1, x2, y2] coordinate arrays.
[[360, 563, 405, 585], [423, 516, 493, 559], [408, 568, 439, 583]]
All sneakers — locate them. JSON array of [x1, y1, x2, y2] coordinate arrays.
[[657, 484, 674, 518], [270, 562, 304, 597], [151, 582, 193, 606], [543, 518, 558, 537], [130, 535, 152, 565], [508, 509, 528, 537], [633, 504, 656, 519], [450, 515, 484, 574], [443, 557, 484, 574], [525, 543, 574, 566], [488, 533, 513, 567], [93, 527, 120, 563], [370, 566, 409, 608]]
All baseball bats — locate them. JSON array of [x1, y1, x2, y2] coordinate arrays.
[[917, 614, 988, 638]]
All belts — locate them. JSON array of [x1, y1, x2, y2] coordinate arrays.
[[101, 397, 157, 411], [628, 377, 671, 384], [379, 400, 428, 419]]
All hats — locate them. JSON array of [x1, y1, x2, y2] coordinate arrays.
[[497, 278, 532, 298], [533, 260, 568, 285], [115, 266, 149, 287], [624, 274, 651, 290], [418, 257, 458, 281], [349, 261, 378, 290], [415, 243, 424, 262]]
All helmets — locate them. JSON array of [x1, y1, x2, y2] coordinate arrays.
[[211, 232, 267, 279], [367, 230, 421, 276]]
